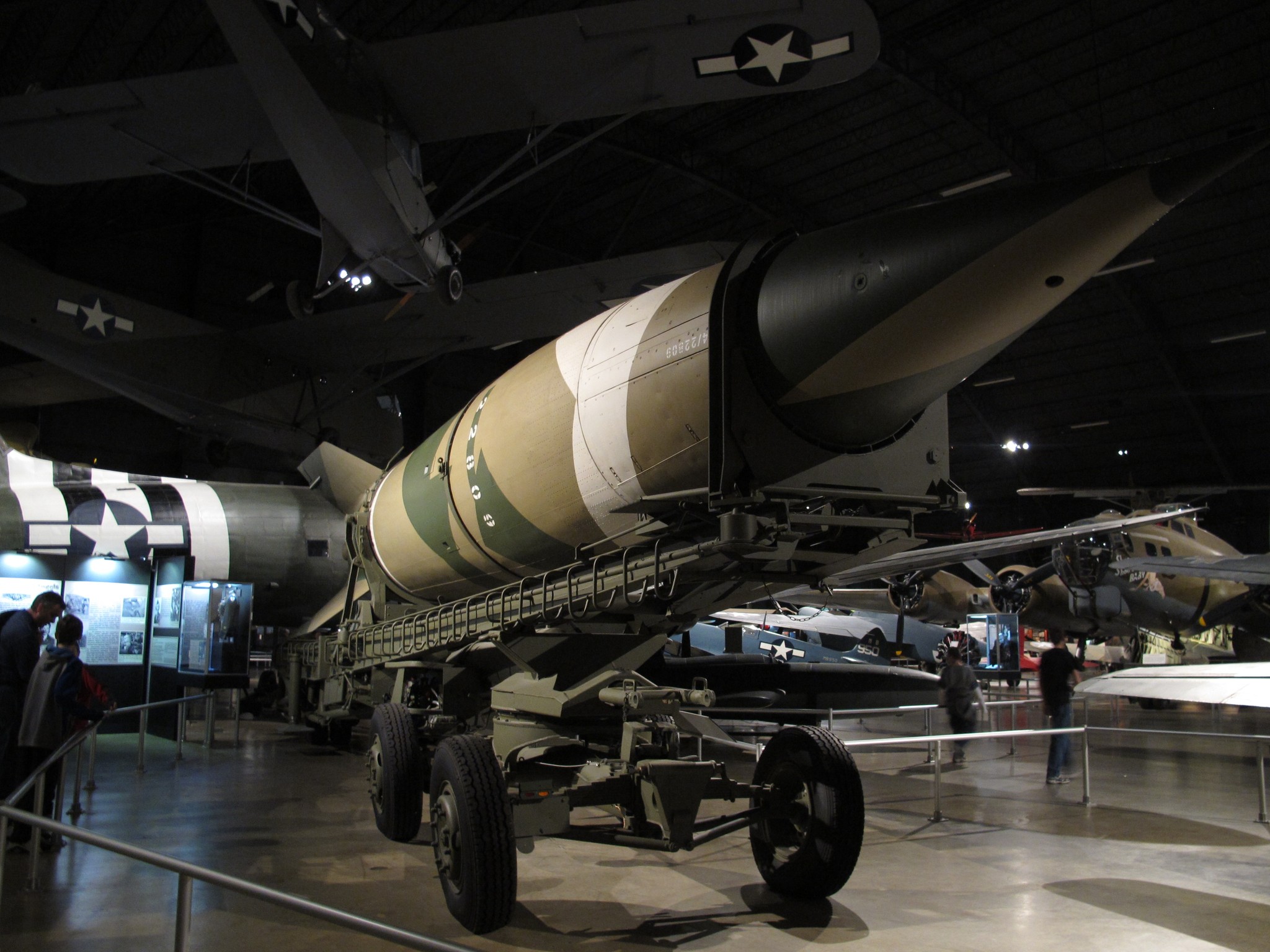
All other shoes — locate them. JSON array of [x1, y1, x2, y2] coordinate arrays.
[[953, 759, 965, 767], [8, 837, 42, 855], [38, 835, 67, 853]]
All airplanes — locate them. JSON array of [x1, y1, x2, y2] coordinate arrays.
[[0, 1, 1270, 939]]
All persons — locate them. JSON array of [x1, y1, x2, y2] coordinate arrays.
[[4, 615, 86, 852], [65, 645, 117, 746], [1035, 627, 1081, 785], [0, 591, 69, 803], [6, 585, 183, 656], [988, 633, 1021, 689], [935, 648, 989, 765]]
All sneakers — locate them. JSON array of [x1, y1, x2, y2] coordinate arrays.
[[1046, 773, 1070, 784]]
